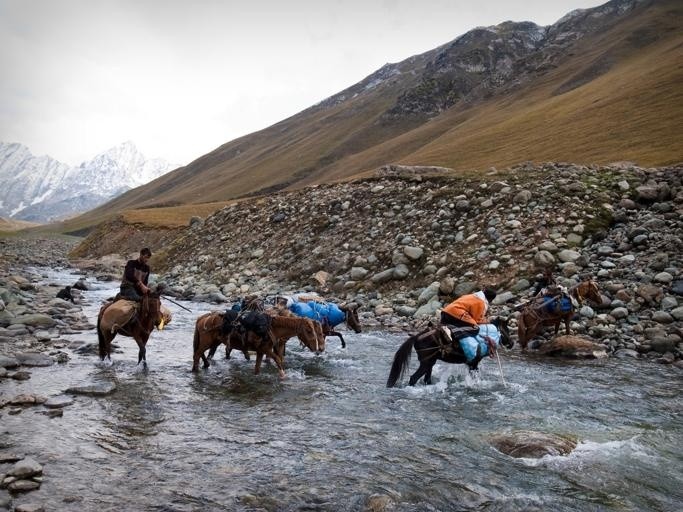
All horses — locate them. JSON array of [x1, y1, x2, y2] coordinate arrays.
[[514, 278, 603, 354], [190, 295, 363, 376], [96, 286, 164, 366], [386, 314, 514, 391]]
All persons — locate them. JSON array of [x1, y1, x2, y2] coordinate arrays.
[[440, 287, 496, 343], [530, 271, 556, 302], [120, 247, 171, 331]]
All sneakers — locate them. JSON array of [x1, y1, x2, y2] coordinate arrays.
[[114, 293, 122, 303], [441, 326, 453, 343]]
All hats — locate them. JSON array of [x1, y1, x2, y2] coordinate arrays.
[[484, 288, 496, 300]]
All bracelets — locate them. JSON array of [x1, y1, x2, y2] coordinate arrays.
[[137, 280, 142, 286]]
